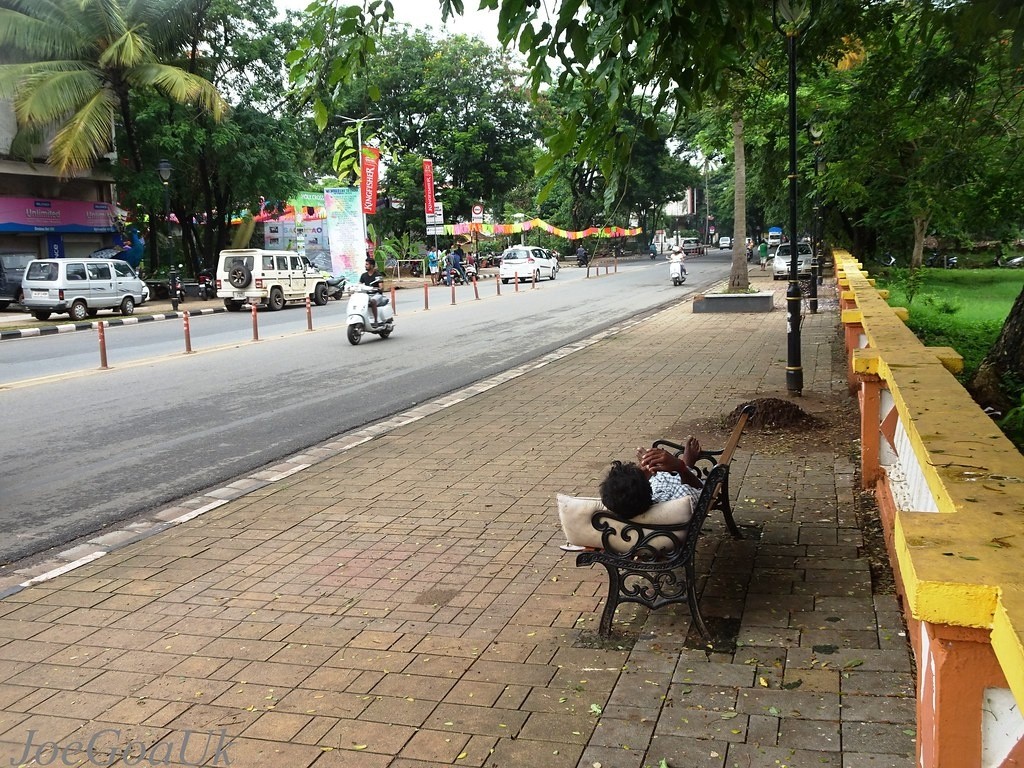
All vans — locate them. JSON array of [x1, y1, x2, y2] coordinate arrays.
[[679, 237, 704, 256], [21, 257, 151, 321], [719, 236, 731, 250]]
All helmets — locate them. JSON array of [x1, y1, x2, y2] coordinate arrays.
[[672, 246, 680, 252]]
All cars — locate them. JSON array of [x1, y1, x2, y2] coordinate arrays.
[[768, 242, 814, 280], [0, 253, 46, 311], [500, 246, 558, 284]]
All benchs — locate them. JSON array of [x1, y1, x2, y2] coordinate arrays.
[[574, 403, 756, 642]]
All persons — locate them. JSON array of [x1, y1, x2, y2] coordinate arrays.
[[348, 258, 383, 328], [745, 239, 754, 253], [757, 239, 771, 271], [650, 243, 657, 256], [669, 246, 686, 278], [547, 250, 561, 268], [600, 436, 705, 520], [576, 245, 588, 268], [427, 245, 505, 286]]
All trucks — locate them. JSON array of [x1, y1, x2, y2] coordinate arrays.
[[767, 227, 783, 249]]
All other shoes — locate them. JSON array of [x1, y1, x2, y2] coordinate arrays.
[[373, 323, 378, 327], [432, 282, 439, 286], [447, 285, 449, 286]]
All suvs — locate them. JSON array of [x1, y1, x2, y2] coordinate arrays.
[[216, 249, 329, 311]]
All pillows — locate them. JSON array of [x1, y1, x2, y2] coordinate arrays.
[[556, 492, 692, 559]]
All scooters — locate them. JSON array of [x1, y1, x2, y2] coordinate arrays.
[[746, 248, 753, 261], [439, 260, 480, 286], [872, 247, 898, 268], [343, 274, 395, 345], [411, 263, 421, 277], [666, 254, 687, 286], [924, 250, 959, 269], [990, 247, 1024, 269]]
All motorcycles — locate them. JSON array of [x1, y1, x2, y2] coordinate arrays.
[[576, 248, 590, 267], [160, 264, 187, 304], [650, 252, 656, 260], [321, 275, 347, 301], [193, 266, 217, 301]]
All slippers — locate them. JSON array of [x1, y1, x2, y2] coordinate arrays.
[[559, 542, 585, 551]]
